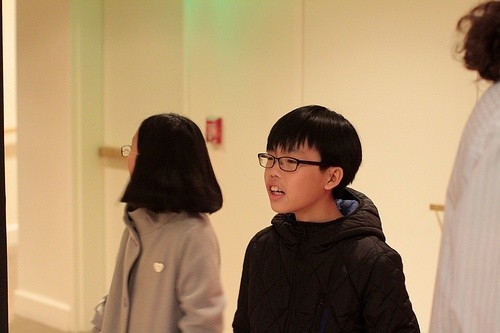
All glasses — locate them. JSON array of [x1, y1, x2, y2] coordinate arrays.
[[120, 144, 139, 158], [257, 153, 320, 172]]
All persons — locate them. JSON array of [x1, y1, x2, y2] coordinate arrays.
[[426, 0, 500, 333], [90, 114, 224, 333], [232, 106, 422, 333]]
